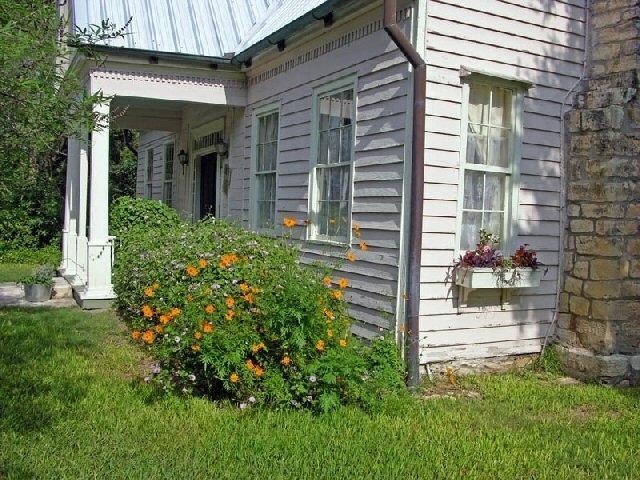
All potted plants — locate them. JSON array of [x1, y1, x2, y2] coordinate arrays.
[[15, 272, 55, 302]]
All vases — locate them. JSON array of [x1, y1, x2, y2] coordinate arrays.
[[453, 267, 543, 290]]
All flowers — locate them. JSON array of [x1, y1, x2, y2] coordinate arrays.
[[445, 230, 549, 274]]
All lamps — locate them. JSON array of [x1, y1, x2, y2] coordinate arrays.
[[176, 149, 189, 175]]
